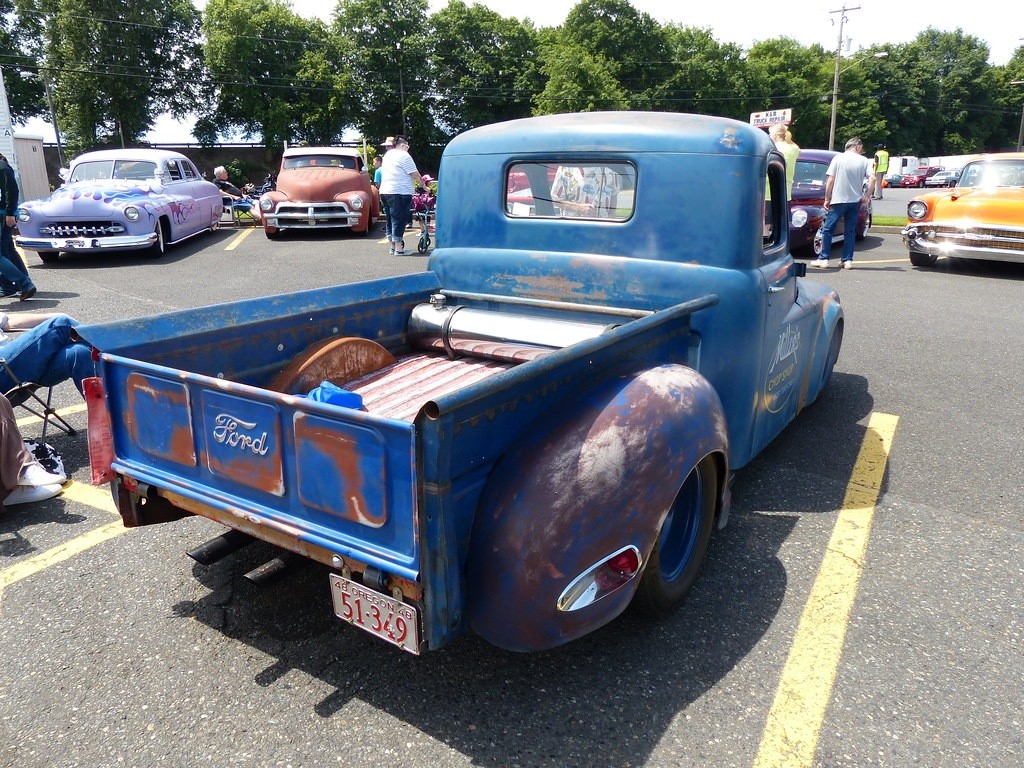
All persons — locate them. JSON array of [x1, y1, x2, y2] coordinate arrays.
[[299, 141, 310, 147], [0, 155, 37, 300], [0, 311, 95, 402], [379, 135, 430, 256], [873, 144, 890, 200], [416, 174, 434, 237], [810, 138, 876, 270], [373, 157, 386, 231], [380, 137, 414, 228], [0, 392, 65, 506], [551, 166, 620, 218], [765, 123, 800, 235], [265, 172, 276, 191], [212, 166, 260, 220]]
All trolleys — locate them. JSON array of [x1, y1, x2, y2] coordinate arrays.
[[401, 189, 437, 255]]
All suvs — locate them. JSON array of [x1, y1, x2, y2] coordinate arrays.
[[901, 167, 945, 188]]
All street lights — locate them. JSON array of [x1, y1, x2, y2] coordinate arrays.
[[828, 51, 888, 149]]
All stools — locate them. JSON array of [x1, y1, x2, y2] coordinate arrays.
[[222, 196, 256, 228], [0, 357, 78, 458]]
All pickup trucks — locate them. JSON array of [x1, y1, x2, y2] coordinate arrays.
[[69, 110, 845, 658]]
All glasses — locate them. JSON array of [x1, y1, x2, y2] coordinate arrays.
[[401, 144, 410, 149]]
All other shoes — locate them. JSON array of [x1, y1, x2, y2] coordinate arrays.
[[838, 260, 852, 269], [390, 247, 395, 254], [17, 463, 66, 486], [810, 259, 829, 268], [871, 196, 875, 198], [3, 482, 62, 507], [872, 197, 883, 200], [406, 224, 413, 228], [1, 291, 13, 298], [382, 225, 387, 230], [416, 230, 425, 236], [20, 286, 36, 300], [394, 250, 413, 255]]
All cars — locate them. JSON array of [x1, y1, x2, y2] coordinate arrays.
[[259, 147, 381, 240], [882, 170, 961, 188], [15, 149, 222, 264], [764, 149, 872, 256], [901, 152, 1024, 266]]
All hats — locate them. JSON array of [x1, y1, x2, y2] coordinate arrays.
[[381, 137, 394, 146], [423, 174, 435, 181], [878, 144, 886, 149]]
